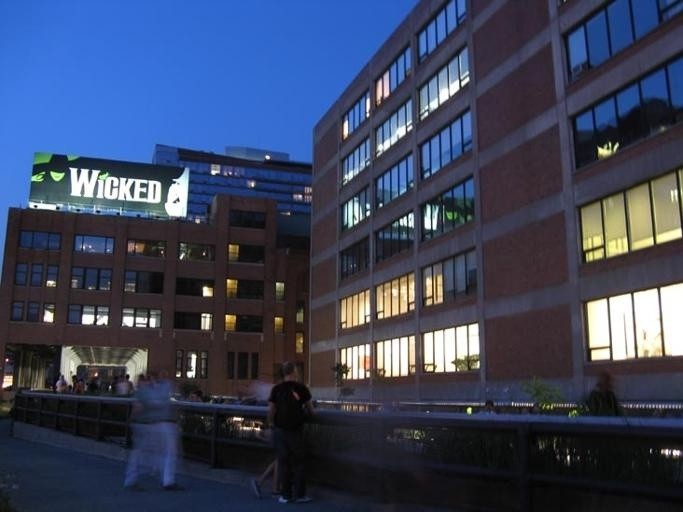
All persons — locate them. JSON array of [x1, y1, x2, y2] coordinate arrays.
[[51, 371, 207, 405], [585, 364, 630, 419], [478, 399, 498, 416], [263, 361, 320, 504], [122, 365, 188, 493], [251, 433, 279, 499]]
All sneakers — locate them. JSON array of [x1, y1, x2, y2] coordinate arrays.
[[252, 480, 315, 503], [123, 480, 185, 490]]
